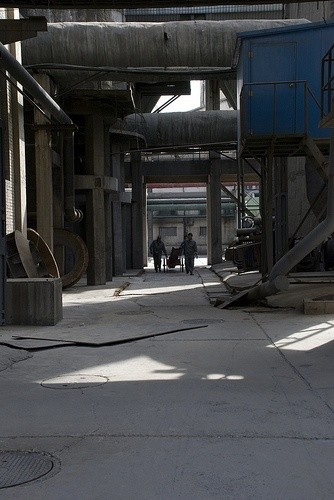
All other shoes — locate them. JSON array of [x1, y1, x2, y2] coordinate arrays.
[[155, 265, 162, 272], [186, 269, 195, 275]]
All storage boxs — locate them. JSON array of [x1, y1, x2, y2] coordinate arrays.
[[4, 278, 63, 327]]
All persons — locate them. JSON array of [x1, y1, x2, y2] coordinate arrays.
[[179, 233, 198, 275], [149, 235, 168, 273]]
